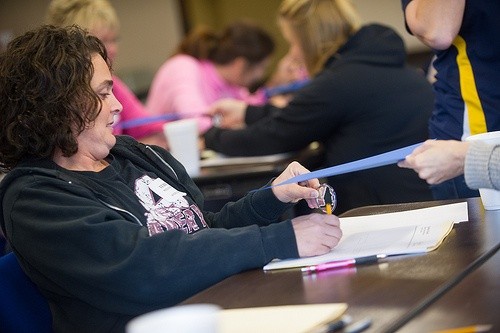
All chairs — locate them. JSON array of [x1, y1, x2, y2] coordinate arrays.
[[1, 252, 51, 333]]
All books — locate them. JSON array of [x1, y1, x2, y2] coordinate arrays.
[[263, 219, 455, 271]]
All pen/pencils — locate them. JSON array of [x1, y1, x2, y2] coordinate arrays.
[[300, 254, 387, 272], [324, 187, 332, 215], [347, 321, 372, 333], [316, 315, 351, 333], [437, 324, 493, 333]]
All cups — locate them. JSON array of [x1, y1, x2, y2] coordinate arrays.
[[164, 118, 200, 177], [465, 130, 500, 211]]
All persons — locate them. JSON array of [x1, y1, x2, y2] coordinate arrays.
[[145, 22, 276, 132], [0, 23, 341, 333], [398, 141, 500, 191], [200, 0, 432, 219], [401, 0, 500, 200], [48, 0, 165, 148]]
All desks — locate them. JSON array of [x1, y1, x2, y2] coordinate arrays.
[[394, 250, 500, 333], [184, 140, 315, 214], [175, 191, 500, 333]]
